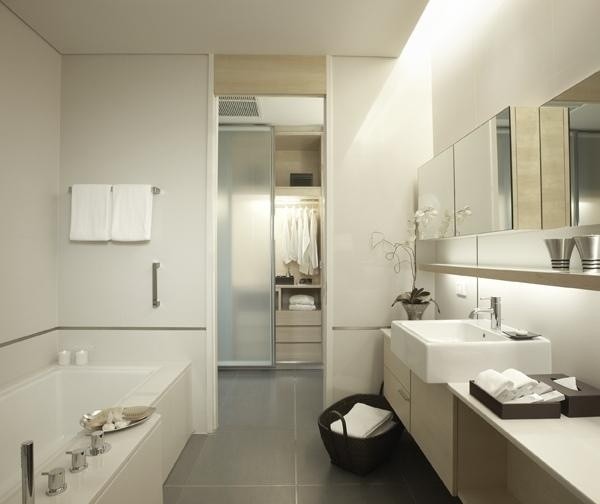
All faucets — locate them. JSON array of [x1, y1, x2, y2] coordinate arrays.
[[468, 297, 502, 329]]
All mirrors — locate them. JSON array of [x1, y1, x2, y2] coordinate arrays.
[[539, 70, 600, 229]]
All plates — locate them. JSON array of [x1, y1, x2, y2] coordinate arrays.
[[81, 404, 153, 432], [502, 328, 542, 340]]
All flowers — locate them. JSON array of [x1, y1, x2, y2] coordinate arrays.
[[439, 205, 472, 236]]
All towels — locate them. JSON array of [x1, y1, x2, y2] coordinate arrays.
[[330, 401, 394, 438], [111, 184, 153, 241], [289, 305, 317, 310], [474, 368, 539, 401], [289, 295, 315, 305], [69, 184, 111, 242]]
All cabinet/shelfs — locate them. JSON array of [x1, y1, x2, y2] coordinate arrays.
[[276, 311, 323, 370], [383, 334, 456, 497], [418, 106, 517, 241]]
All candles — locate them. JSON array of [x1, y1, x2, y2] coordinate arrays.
[[58, 351, 71, 365]]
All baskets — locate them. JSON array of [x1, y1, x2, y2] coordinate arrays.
[[318, 380, 406, 478]]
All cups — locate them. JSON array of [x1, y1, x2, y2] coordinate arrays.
[[58, 349, 71, 367], [543, 237, 576, 270], [573, 234, 600, 272]]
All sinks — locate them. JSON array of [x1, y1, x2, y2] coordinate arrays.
[[390, 318, 552, 384]]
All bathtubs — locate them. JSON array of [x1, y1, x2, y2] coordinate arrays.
[[0, 360, 193, 504]]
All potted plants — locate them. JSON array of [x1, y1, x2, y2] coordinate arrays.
[[391, 288, 440, 320]]
